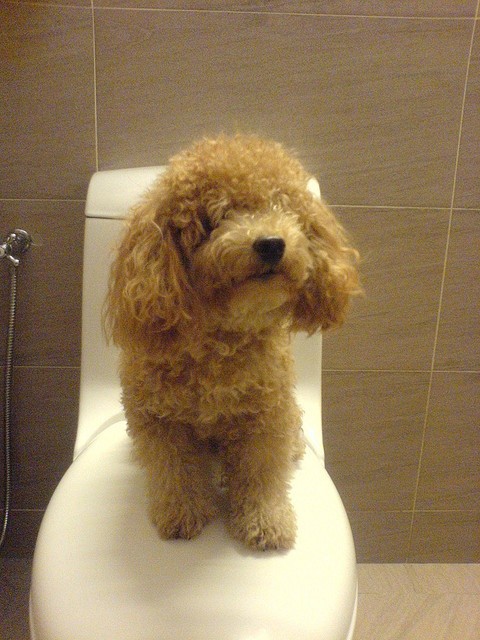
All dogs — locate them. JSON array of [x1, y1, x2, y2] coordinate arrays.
[[100, 129, 367, 556]]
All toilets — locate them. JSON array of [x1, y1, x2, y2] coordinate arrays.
[[28, 166, 358, 640]]
